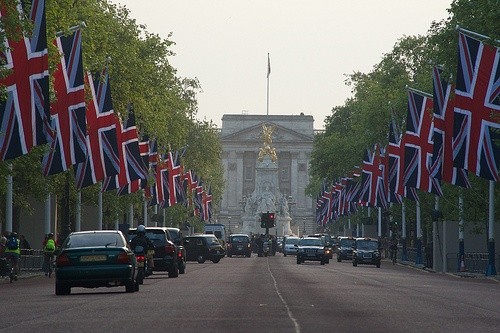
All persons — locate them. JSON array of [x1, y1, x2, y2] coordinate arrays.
[[0, 237, 7, 278], [388, 241, 397, 263], [4, 232, 20, 281], [129, 225, 155, 276], [42, 233, 56, 275], [253, 234, 277, 257], [0, 232, 33, 255]]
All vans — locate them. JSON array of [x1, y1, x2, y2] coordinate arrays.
[[284, 236, 301, 257], [183, 234, 226, 263]]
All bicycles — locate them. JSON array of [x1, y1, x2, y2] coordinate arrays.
[[53, 230, 139, 296], [40, 252, 54, 279], [6, 255, 15, 283]]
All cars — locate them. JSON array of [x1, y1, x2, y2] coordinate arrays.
[[277, 233, 356, 262], [167, 228, 187, 274], [127, 227, 179, 278]]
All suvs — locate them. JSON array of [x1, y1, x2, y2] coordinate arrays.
[[293, 235, 326, 266], [352, 237, 383, 268], [227, 233, 253, 258]]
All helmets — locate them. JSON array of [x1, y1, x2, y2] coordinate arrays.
[[11, 232, 18, 236], [48, 232, 54, 236], [136, 224, 146, 234]]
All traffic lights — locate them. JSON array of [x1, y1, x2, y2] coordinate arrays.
[[260, 212, 267, 229], [268, 213, 275, 228]]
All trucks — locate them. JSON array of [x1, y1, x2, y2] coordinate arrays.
[[203, 223, 227, 247]]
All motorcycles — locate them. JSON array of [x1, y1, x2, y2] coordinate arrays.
[[128, 239, 151, 285]]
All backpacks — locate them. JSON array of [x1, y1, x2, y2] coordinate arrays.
[[45, 239, 55, 250], [7, 237, 18, 250]]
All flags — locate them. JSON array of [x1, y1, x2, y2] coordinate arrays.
[[146, 152, 212, 222], [41, 22, 87, 178], [0, 0, 52, 160], [433, 66, 471, 190], [316, 161, 374, 226], [117, 141, 148, 196], [452, 26, 500, 183], [374, 142, 387, 207], [73, 68, 120, 190], [387, 102, 404, 204], [102, 103, 145, 192], [403, 85, 443, 198]]
